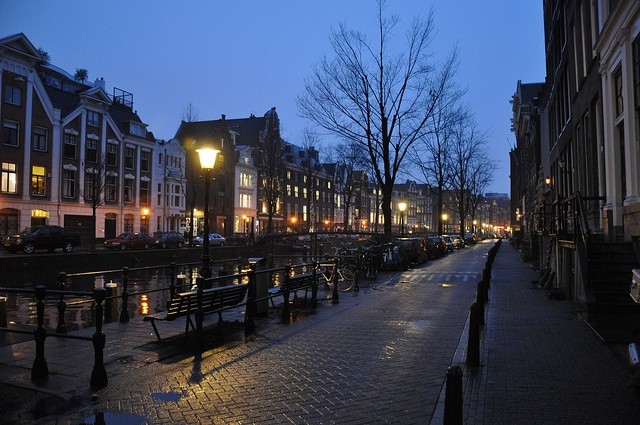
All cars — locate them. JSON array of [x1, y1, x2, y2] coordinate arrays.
[[104, 233, 156, 250], [390, 238, 427, 268], [450, 234, 465, 249], [193, 233, 226, 247], [155, 232, 184, 248], [440, 234, 454, 252], [4, 226, 80, 254]]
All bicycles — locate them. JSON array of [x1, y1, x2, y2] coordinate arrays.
[[301, 260, 354, 292], [331, 237, 389, 279]]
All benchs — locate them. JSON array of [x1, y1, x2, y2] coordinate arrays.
[[267, 270, 328, 309], [143, 283, 249, 345]]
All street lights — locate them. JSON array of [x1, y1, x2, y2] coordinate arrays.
[[397, 201, 407, 237], [195, 143, 220, 273]]
